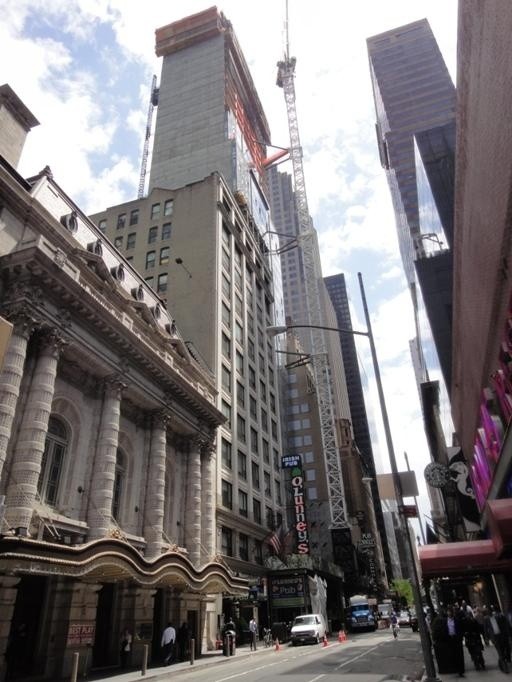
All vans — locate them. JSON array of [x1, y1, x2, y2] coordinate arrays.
[[290, 614, 327, 643]]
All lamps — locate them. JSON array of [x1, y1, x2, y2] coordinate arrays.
[[8, 526, 28, 537], [55, 534, 71, 545]]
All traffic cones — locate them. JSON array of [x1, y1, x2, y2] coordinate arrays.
[[338, 630, 347, 642], [274, 638, 281, 652], [323, 635, 328, 647]]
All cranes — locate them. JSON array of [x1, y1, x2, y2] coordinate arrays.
[[271, 0, 362, 610]]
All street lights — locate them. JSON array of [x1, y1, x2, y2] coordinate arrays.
[[362, 474, 389, 591], [266, 269, 438, 681]]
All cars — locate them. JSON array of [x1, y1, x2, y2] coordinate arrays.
[[377, 600, 417, 628]]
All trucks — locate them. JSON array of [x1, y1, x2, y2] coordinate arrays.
[[345, 595, 377, 633]]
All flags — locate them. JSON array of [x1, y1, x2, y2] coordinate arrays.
[[270, 525, 284, 555]]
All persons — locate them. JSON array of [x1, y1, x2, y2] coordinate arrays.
[[118, 626, 134, 669], [160, 621, 177, 665], [440, 599, 511, 673], [248, 616, 259, 651], [388, 607, 400, 633], [179, 621, 193, 662]]
